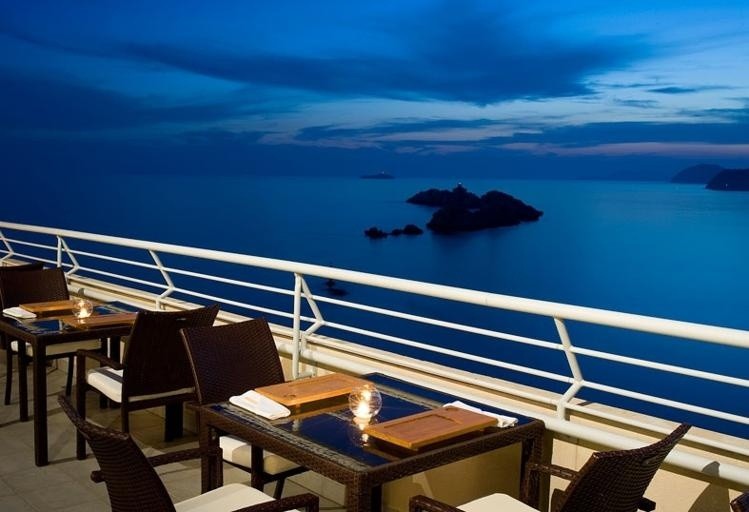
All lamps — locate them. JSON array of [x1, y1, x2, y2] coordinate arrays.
[[72, 299, 94, 320], [349, 385, 382, 419]]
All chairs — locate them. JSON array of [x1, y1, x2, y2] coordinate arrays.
[[2, 267, 109, 422], [408, 421, 693, 512], [180, 318, 314, 500], [75, 305, 219, 459], [56, 392, 319, 512], [1, 262, 57, 274], [731, 492, 748, 512]]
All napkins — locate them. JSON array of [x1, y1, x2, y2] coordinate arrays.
[[444, 400, 519, 428], [228, 390, 292, 421], [3, 306, 36, 319]]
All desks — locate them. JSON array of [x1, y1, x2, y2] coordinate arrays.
[[1, 302, 149, 466], [198, 374, 545, 512]]
